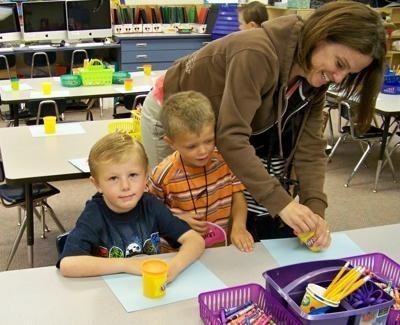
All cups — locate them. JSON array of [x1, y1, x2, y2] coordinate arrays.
[[300, 284, 339, 316]]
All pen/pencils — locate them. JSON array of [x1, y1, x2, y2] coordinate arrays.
[[364, 271, 400, 310], [324, 260, 373, 301], [220, 300, 276, 324], [392, 65, 400, 76]]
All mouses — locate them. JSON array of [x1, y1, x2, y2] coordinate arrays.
[[104, 41, 110, 45], [64, 42, 70, 47]]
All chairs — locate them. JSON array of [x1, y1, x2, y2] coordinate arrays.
[[61, 48, 101, 75], [22, 49, 59, 77], [1, 161, 70, 268], [22, 99, 70, 125], [0, 53, 18, 79], [110, 93, 150, 118], [325, 99, 400, 192]]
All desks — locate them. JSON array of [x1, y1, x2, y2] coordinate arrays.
[[0, 221, 400, 325], [115, 33, 212, 72], [0, 68, 169, 124], [326, 82, 400, 192], [0, 117, 143, 268], [0, 40, 122, 55]]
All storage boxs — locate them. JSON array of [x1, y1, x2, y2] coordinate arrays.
[[343, 253, 400, 325], [60, 75, 81, 87], [131, 109, 143, 120], [262, 259, 396, 325], [79, 59, 113, 86], [381, 75, 400, 95], [198, 283, 301, 325], [112, 71, 131, 84], [107, 120, 143, 141]]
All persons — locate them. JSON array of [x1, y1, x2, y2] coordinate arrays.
[[56, 133, 205, 285], [238, 1, 269, 30], [140, 1, 387, 249], [145, 91, 255, 252]]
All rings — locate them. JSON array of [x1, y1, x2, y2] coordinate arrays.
[[326, 230, 330, 233]]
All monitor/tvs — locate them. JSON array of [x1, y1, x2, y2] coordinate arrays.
[[21, 0, 68, 44], [66, 0, 113, 42], [0, 2, 23, 42]]
